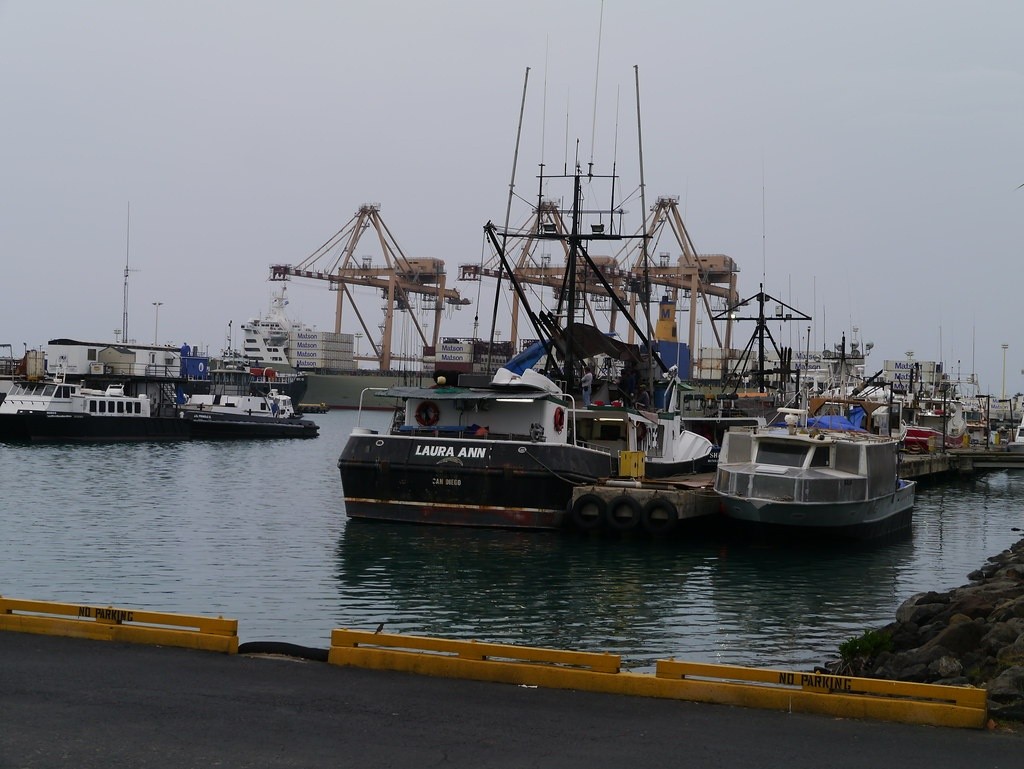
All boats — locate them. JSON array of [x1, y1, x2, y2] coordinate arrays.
[[0, 200, 322, 446]]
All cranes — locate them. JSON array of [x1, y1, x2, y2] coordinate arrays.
[[454, 193, 629, 362], [275, 201, 462, 371], [607, 192, 742, 385]]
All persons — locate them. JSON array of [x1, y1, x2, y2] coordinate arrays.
[[580, 366, 593, 408], [630, 383, 651, 412]]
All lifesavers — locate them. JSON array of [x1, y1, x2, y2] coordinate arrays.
[[895, 479, 904, 489], [555, 407, 564, 432], [625, 421, 647, 442], [415, 401, 440, 426]]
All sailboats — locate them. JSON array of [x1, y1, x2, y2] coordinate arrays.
[[696, 267, 1024, 541], [333, 0, 712, 524]]
